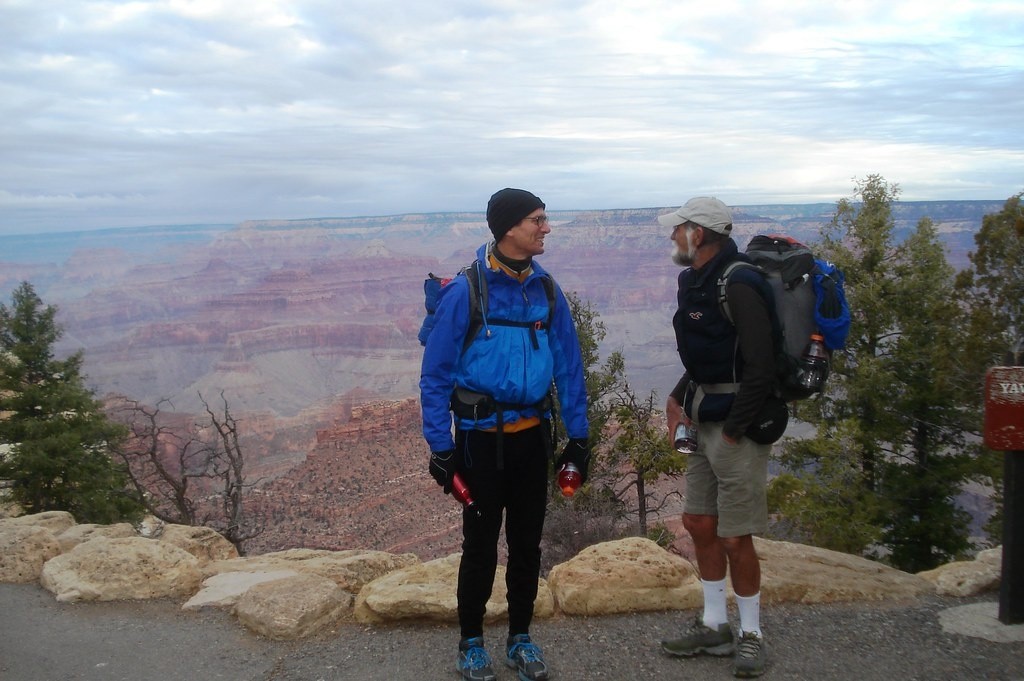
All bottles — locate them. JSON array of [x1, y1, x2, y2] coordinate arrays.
[[800, 334, 828, 388], [450, 468, 482, 518], [558, 447, 583, 498], [675, 407, 698, 454]]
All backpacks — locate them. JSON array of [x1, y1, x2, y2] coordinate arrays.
[[718, 235, 852, 404], [417, 264, 557, 351]]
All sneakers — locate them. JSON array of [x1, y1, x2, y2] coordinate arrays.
[[661, 620, 733, 655], [456, 637, 497, 681], [506, 633, 549, 681], [732, 629, 765, 675]]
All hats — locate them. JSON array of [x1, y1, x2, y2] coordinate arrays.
[[658, 195, 732, 235], [486, 188, 545, 241]]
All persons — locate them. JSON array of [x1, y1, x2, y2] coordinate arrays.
[[419, 186, 591, 680], [659, 194, 788, 678]]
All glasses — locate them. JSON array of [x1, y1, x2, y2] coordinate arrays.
[[672, 225, 690, 232], [524, 215, 549, 228]]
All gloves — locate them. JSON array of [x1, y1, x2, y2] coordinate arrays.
[[428, 450, 462, 495], [558, 437, 591, 486]]
